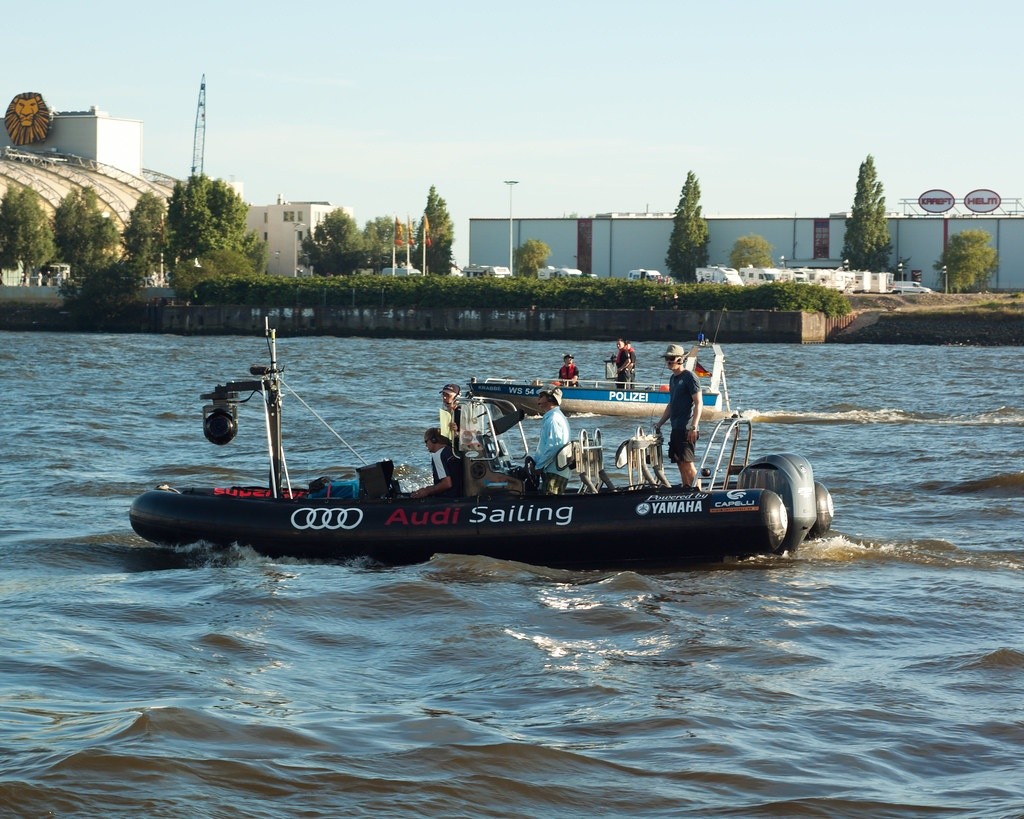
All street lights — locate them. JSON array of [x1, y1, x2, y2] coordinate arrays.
[[504, 180, 518, 273]]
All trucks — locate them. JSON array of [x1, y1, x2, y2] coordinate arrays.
[[462, 264, 510, 279], [739, 263, 894, 293], [537, 266, 583, 281], [695, 265, 744, 286]]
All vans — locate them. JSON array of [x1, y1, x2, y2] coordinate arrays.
[[627, 270, 666, 281], [894, 281, 931, 294], [383, 268, 419, 276]]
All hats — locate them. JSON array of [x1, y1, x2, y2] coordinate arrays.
[[660, 345, 684, 357], [564, 354, 574, 359], [536, 384, 563, 406], [439, 384, 460, 396]]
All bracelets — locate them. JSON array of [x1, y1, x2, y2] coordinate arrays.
[[688, 426, 699, 431]]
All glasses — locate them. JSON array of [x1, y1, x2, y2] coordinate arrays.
[[425, 438, 430, 444], [665, 356, 681, 362], [539, 394, 547, 398]]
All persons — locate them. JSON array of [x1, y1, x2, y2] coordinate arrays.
[[530, 383, 572, 496], [652, 344, 703, 491], [410, 428, 465, 499], [559, 354, 579, 387], [616, 337, 636, 389], [438, 384, 461, 440]]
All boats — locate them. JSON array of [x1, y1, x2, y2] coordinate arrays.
[[130, 318, 834, 566], [467, 345, 739, 421]]
